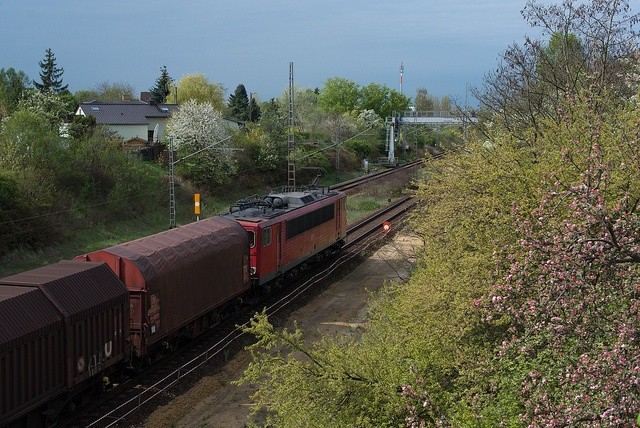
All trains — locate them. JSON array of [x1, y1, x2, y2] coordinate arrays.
[[0, 185, 347, 428]]
